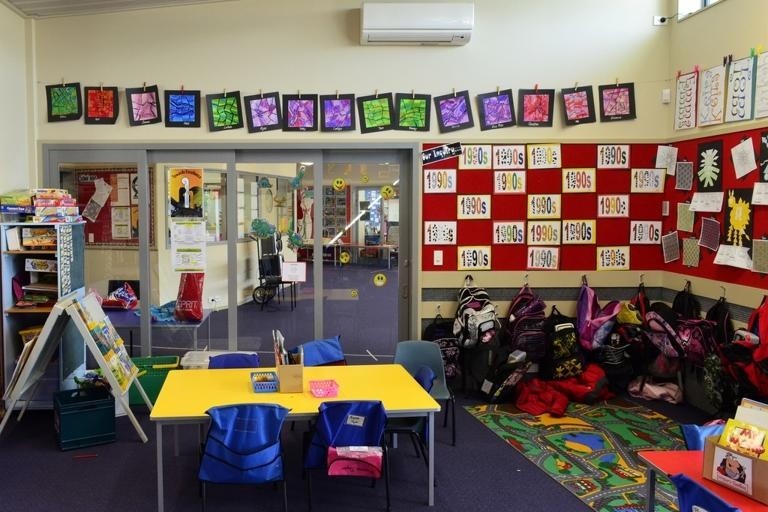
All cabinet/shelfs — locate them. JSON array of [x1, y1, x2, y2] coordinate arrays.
[[294, 183, 354, 264], [0, 215, 89, 415]]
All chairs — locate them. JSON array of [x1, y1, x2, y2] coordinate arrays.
[[284, 332, 348, 433], [368, 363, 442, 490], [104, 277, 140, 358], [297, 398, 401, 512], [192, 401, 296, 510], [390, 338, 458, 447], [667, 466, 741, 512], [257, 255, 298, 313], [676, 416, 728, 451], [269, 327, 305, 432], [196, 350, 263, 452]]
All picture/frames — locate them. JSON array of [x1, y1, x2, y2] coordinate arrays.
[[55, 162, 160, 254]]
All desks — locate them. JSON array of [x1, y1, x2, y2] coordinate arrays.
[[143, 360, 446, 512], [96, 304, 216, 363], [330, 240, 401, 271], [634, 450, 768, 512], [297, 237, 354, 270]]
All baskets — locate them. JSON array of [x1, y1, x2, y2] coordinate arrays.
[[251, 371, 280, 393], [311, 380, 338, 398], [127, 356, 180, 405]]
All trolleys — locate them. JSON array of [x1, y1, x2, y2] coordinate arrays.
[[248, 231, 282, 304]]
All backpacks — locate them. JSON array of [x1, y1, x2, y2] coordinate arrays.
[[423, 274, 582, 404], [576, 275, 768, 413]]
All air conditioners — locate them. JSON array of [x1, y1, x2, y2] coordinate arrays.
[[356, 1, 476, 49]]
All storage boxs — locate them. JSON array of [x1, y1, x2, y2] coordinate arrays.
[[177, 347, 259, 371], [697, 435, 768, 509], [118, 353, 180, 407], [51, 383, 119, 454]]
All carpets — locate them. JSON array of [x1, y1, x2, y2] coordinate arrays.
[[454, 388, 697, 512]]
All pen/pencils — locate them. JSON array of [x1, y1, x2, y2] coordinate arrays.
[[74, 455, 98, 457]]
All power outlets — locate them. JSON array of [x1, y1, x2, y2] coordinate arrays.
[[651, 15, 671, 27]]
